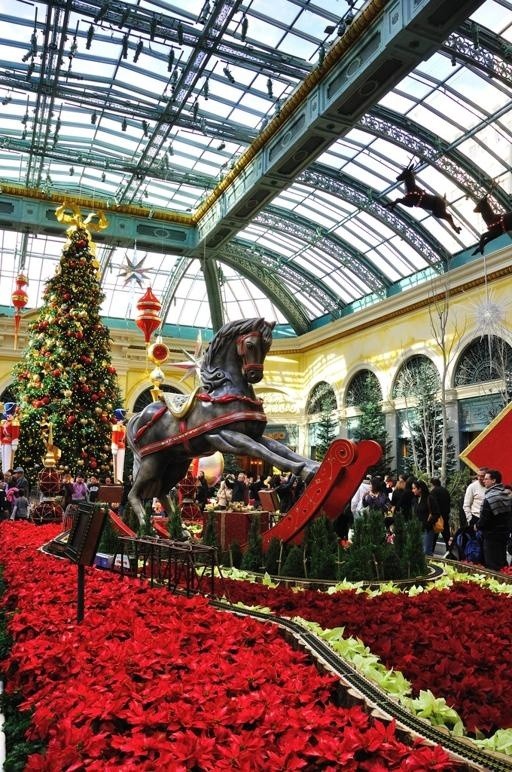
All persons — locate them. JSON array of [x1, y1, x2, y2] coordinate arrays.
[[0, 467, 132, 522], [351, 465, 512, 573], [197, 470, 303, 510]]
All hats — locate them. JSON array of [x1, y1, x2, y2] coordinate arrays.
[[14, 466, 25, 473]]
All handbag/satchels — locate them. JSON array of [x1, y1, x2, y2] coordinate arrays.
[[428, 514, 445, 533]]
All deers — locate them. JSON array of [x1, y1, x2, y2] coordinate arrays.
[[382, 151, 462, 234], [471, 178, 512, 256]]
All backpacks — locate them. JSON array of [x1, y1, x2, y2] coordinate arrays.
[[463, 531, 482, 563]]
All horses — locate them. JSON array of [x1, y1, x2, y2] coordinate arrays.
[[125, 317, 323, 543]]
